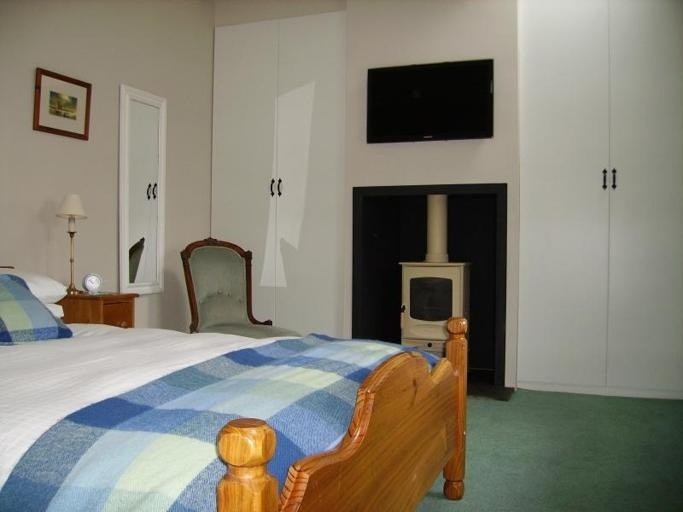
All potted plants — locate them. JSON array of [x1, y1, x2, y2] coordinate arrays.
[[33, 67, 92, 140]]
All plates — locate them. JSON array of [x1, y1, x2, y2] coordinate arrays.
[[213, 10, 348, 337], [129, 99, 159, 283], [517, 0, 683, 400]]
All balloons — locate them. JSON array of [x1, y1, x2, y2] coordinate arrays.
[[83, 272, 103, 295]]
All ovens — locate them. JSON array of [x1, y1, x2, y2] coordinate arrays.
[[0, 265, 468, 512]]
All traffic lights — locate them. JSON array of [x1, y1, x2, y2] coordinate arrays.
[[43, 303, 64, 320], [5, 272, 68, 303], [0, 274, 73, 345]]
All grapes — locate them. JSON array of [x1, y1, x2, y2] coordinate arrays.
[[59, 292, 140, 328]]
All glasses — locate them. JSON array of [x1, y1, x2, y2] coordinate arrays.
[[56, 193, 88, 295]]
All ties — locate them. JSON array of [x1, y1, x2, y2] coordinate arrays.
[[366, 58, 494, 144]]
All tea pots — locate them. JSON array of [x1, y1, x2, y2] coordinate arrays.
[[119, 83, 165, 294]]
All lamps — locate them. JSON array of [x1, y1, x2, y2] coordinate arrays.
[[129, 237, 145, 283], [179, 236, 301, 339]]
